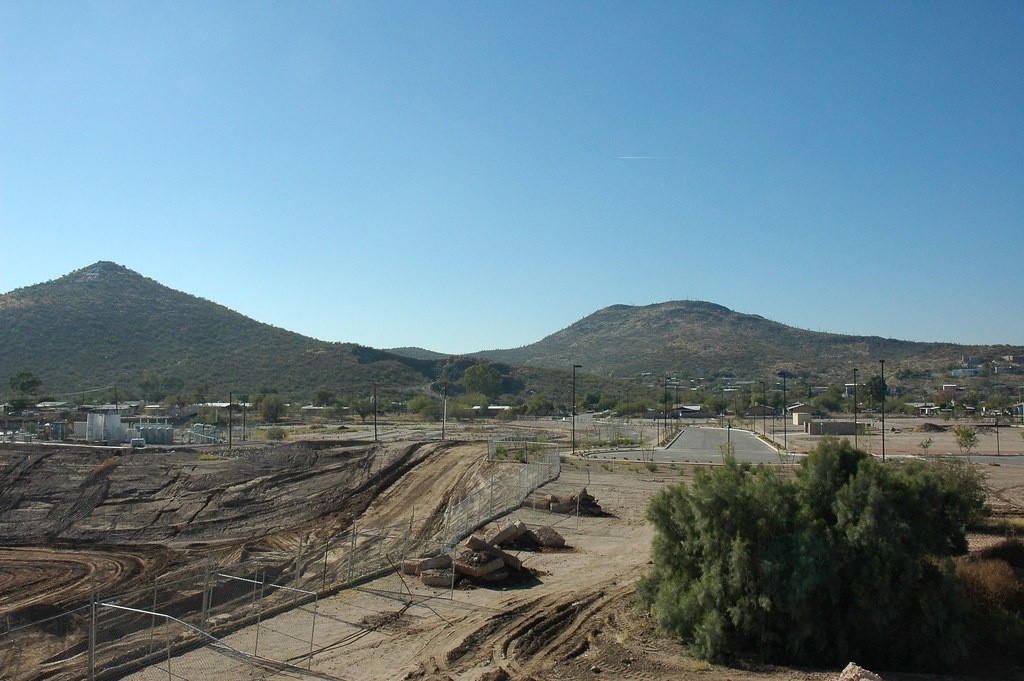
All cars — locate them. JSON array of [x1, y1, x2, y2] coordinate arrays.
[[9, 412, 16, 416]]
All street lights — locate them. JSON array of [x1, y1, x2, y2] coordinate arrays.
[[665, 377, 671, 440], [727, 423, 731, 464], [759, 382, 765, 436], [879, 359, 885, 462], [572, 365, 583, 453], [778, 372, 787, 449], [854, 369, 858, 449]]
